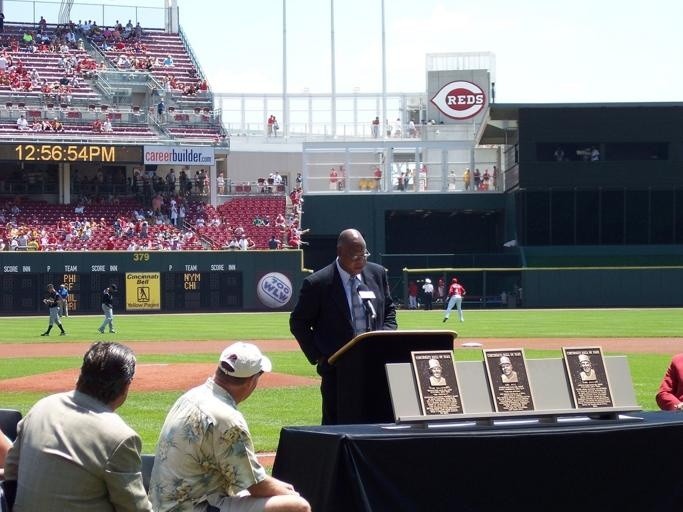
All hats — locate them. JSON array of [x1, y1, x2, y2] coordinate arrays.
[[217, 341, 274, 380]]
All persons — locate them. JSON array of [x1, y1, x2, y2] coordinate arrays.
[[656, 353, 683, 412], [428, 353, 598, 385], [394, 118, 401, 137], [463, 168, 471, 190], [441, 276, 466, 324], [1, 10, 211, 135], [0, 168, 309, 252], [6, 341, 153, 512], [386, 120, 393, 137], [473, 166, 497, 192], [329, 168, 338, 191], [375, 168, 382, 192], [448, 170, 457, 190], [409, 119, 417, 137], [407, 281, 417, 310], [1, 428, 13, 503], [267, 115, 277, 136], [422, 278, 434, 310], [288, 229, 398, 424], [373, 117, 379, 137], [147, 343, 311, 511], [41, 282, 67, 335], [58, 284, 69, 316], [398, 172, 410, 191], [98, 285, 118, 333]]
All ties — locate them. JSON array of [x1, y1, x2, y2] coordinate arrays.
[[349, 275, 367, 338]]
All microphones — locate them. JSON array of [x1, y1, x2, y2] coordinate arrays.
[[357, 287, 376, 319]]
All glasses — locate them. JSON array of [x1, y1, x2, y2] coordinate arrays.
[[347, 248, 371, 262]]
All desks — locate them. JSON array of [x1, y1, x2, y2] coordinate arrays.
[[277, 411, 682, 511]]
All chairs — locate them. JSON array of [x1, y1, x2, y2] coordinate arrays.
[[125, 448, 173, 511], [0, 404, 36, 482], [1, 23, 218, 138], [1, 193, 285, 252]]
